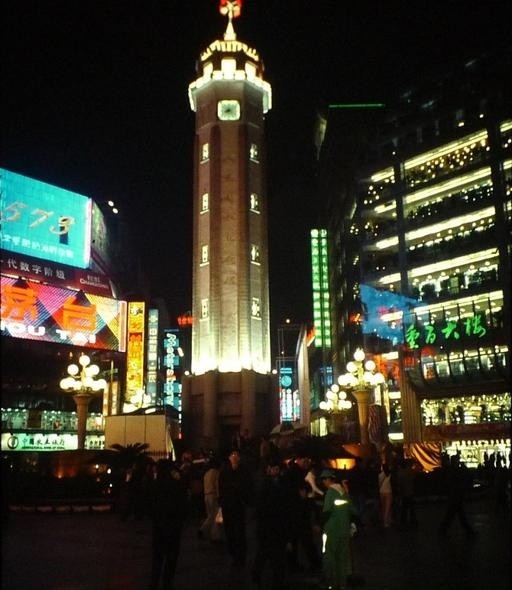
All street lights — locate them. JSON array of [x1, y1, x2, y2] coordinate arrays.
[[55, 352, 111, 452], [314, 383, 354, 436], [334, 343, 388, 446]]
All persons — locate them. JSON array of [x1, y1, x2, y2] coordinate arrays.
[[143, 458, 182, 590], [181, 437, 512, 589]]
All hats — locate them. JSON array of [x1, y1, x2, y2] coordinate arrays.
[[314, 469, 338, 480]]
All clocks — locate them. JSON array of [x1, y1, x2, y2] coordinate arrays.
[[217, 98, 242, 123]]
